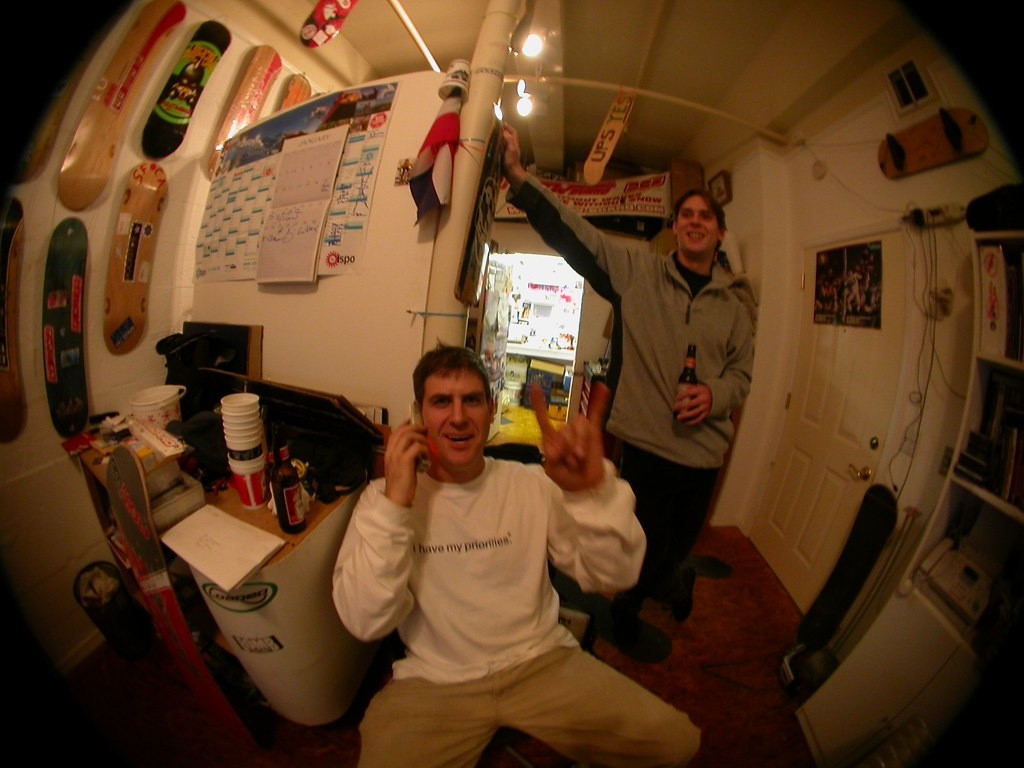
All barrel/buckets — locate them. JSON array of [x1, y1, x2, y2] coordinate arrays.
[[128, 384, 186, 430]]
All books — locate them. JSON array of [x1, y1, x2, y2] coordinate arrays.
[[160, 505, 285, 592]]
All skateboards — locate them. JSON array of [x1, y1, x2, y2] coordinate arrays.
[[793, 484, 900, 661], [1, 0, 356, 448], [877, 105, 990, 181], [583, 91, 640, 186], [103, 445, 261, 756]]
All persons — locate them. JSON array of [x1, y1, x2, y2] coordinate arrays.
[[501, 121, 755, 658], [332, 343, 700, 767]]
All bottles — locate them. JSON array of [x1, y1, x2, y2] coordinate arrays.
[[672, 343, 698, 420], [270, 421, 306, 534]]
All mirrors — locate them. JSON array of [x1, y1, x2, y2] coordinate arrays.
[[0, 0, 1023, 767]]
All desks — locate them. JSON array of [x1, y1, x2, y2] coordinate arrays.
[[77, 422, 376, 725]]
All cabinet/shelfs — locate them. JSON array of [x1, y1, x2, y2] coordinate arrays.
[[795, 583, 977, 768], [578, 361, 607, 418], [904, 228, 1021, 644]]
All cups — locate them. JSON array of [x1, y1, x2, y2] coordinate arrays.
[[438, 58, 471, 101], [220, 393, 268, 510]]
